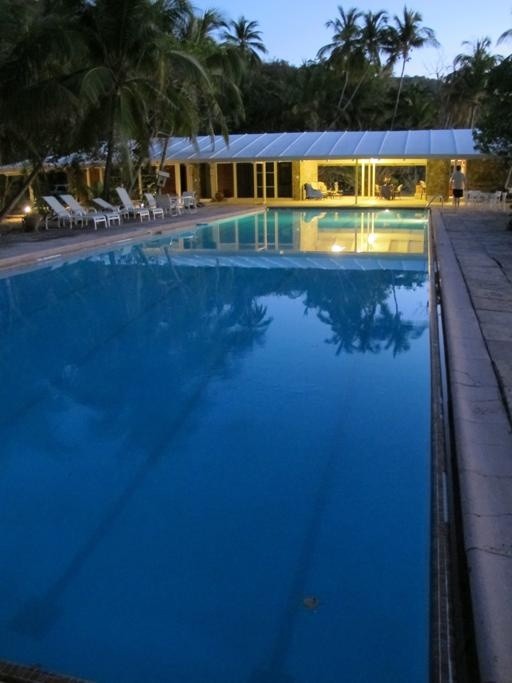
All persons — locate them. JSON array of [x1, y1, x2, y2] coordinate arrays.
[[449, 163, 466, 208]]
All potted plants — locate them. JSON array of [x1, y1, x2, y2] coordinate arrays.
[[22, 214, 37, 232]]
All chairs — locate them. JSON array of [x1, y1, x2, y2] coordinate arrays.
[[463, 190, 508, 213], [41, 186, 198, 230], [304, 181, 334, 201], [374, 183, 403, 200]]
